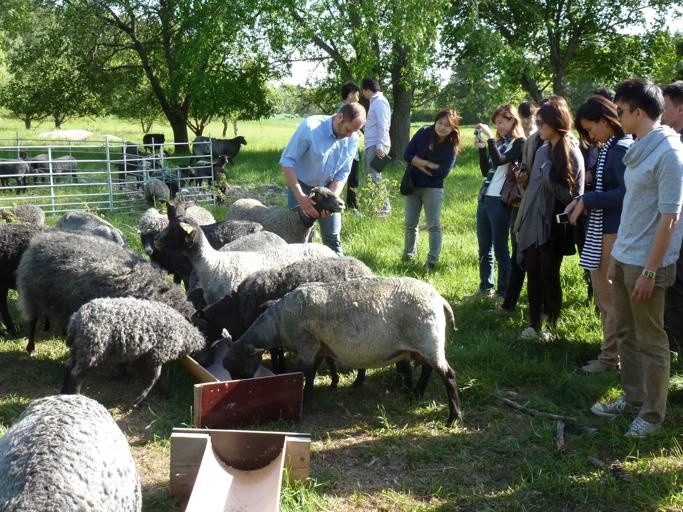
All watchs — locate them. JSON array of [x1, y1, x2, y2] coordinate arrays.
[[643, 270, 656, 278]]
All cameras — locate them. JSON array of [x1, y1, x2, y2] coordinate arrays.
[[510, 159, 527, 173], [556, 213, 569, 224], [473, 129, 481, 140]]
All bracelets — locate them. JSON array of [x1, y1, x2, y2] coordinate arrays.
[[425, 160, 431, 166]]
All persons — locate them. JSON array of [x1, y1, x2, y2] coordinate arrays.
[[399, 108, 460, 272], [592, 77, 683, 442], [278, 101, 366, 258], [660, 81, 682, 344], [335, 83, 364, 221], [474, 95, 635, 376], [360, 76, 393, 218]]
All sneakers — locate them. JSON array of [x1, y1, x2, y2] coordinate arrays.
[[590, 399, 638, 417], [517, 327, 561, 344], [461, 288, 495, 302], [573, 359, 618, 376], [624, 416, 662, 439]]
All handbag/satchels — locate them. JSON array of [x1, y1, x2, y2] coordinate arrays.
[[370, 152, 392, 173], [400, 163, 421, 195], [501, 163, 523, 205]]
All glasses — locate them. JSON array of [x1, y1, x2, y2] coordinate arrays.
[[616, 105, 633, 117], [535, 119, 546, 129]]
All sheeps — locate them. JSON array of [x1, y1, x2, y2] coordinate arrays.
[[0, 151, 79, 196], [0, 187, 415, 410], [223, 275, 464, 428], [114, 134, 248, 206], [0, 394, 144, 512]]
